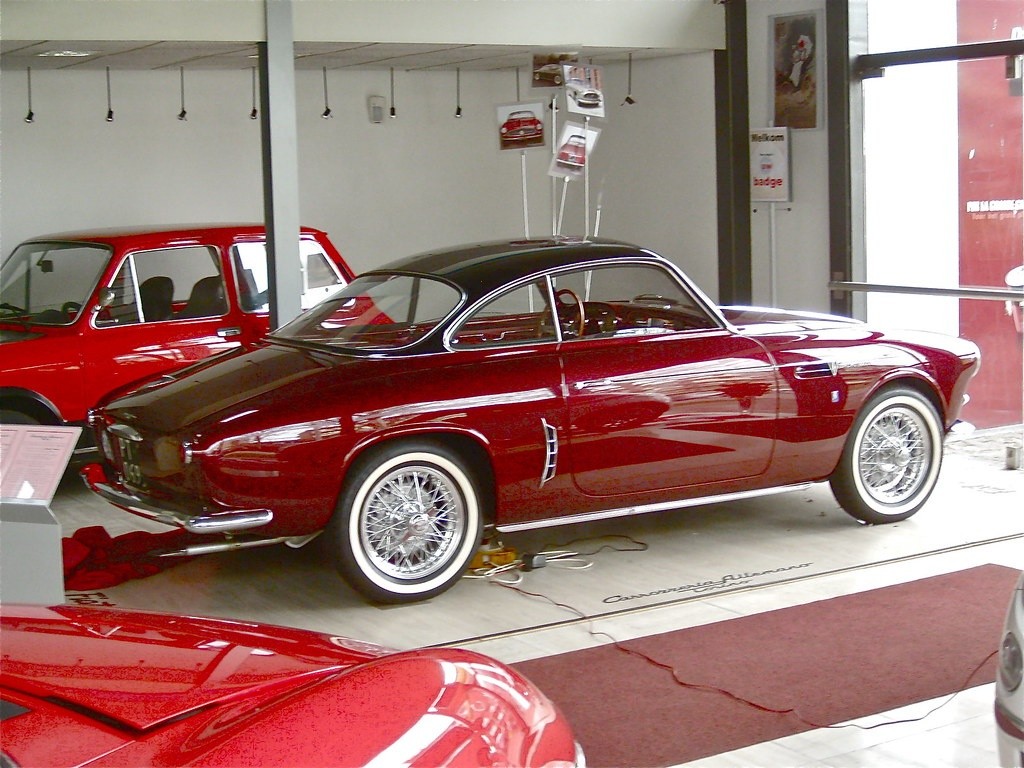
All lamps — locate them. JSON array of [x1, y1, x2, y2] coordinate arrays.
[[388, 67, 397, 117], [625, 52, 635, 105], [321, 67, 331, 118], [23, 66, 34, 123], [455, 67, 461, 118], [176, 64, 187, 121], [548, 103, 560, 112], [250, 65, 257, 119], [105, 65, 113, 122]]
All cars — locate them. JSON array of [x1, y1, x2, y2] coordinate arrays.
[[500, 111, 544, 149], [994, 573, 1024, 768], [556, 135, 585, 170], [80, 236, 982, 605], [1, 222, 396, 454], [566, 78, 601, 108], [533, 64, 564, 85], [0, 601, 586, 768]]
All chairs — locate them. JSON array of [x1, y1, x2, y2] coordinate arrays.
[[118, 277, 175, 323], [175, 276, 227, 320]]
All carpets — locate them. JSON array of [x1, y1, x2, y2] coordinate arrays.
[[509, 562, 1021, 768]]
